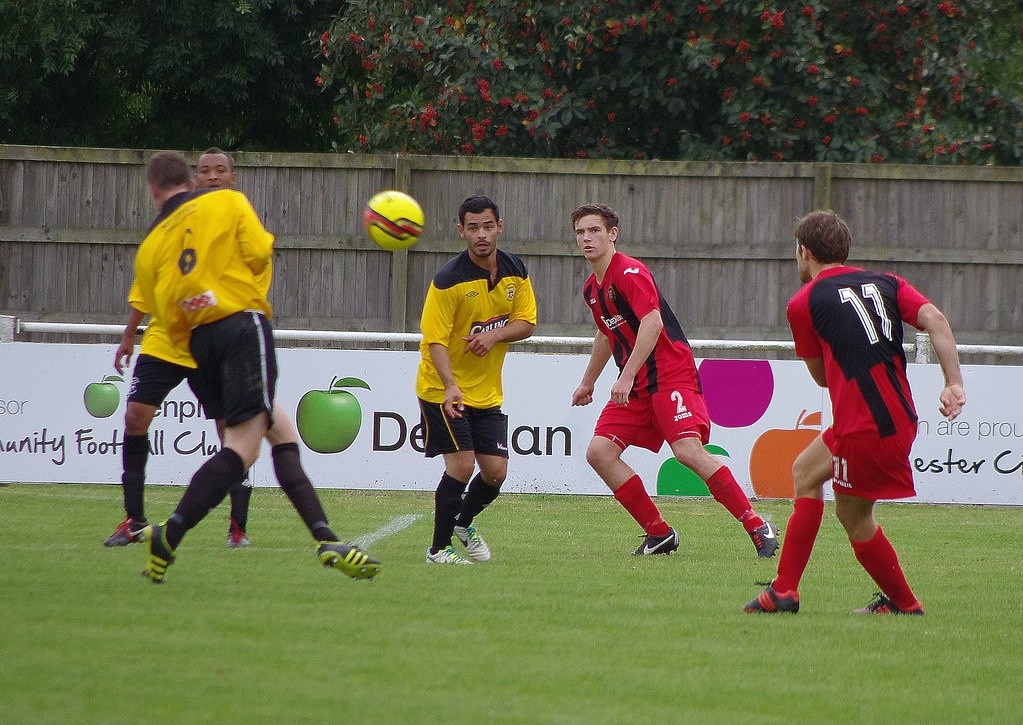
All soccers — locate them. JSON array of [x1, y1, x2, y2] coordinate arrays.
[[362, 189, 426, 252]]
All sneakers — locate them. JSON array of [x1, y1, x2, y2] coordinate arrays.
[[103, 519, 149, 547], [742, 579, 800, 614], [226, 517, 249, 548], [453, 513, 492, 562], [631, 527, 680, 557], [426, 545, 474, 565], [854, 592, 924, 617], [143, 519, 177, 584], [749, 520, 781, 559], [316, 540, 382, 581]]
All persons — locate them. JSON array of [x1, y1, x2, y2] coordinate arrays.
[[744, 209, 967, 618], [570, 205, 780, 558], [413, 196, 537, 566], [114, 152, 381, 585], [104, 147, 256, 547]]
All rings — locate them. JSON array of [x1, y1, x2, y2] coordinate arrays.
[[473, 340, 476, 342], [617, 396, 622, 397]]
